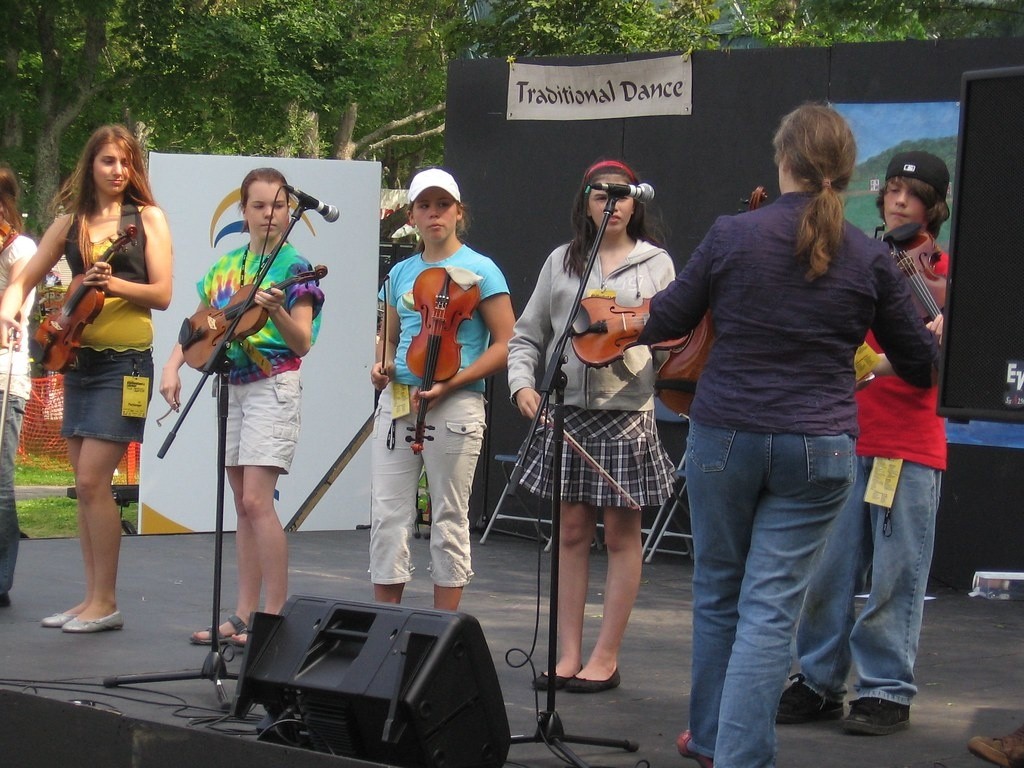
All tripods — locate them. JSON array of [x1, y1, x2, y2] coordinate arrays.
[[103, 202, 308, 715], [505, 195, 639, 768]]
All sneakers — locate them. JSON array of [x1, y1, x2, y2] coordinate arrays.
[[775, 673, 842, 724], [841, 696, 910, 736]]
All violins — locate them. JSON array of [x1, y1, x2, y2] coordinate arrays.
[[178, 264, 329, 372], [29, 224, 138, 374], [655, 184, 769, 419], [570, 296, 692, 368], [875, 222, 947, 321], [404, 266, 481, 456]]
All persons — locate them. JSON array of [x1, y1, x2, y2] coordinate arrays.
[[773, 151, 952, 738], [157, 166, 327, 653], [370, 170, 518, 613], [0, 122, 174, 634], [504, 158, 677, 693], [0, 163, 40, 608], [635, 103, 940, 756]]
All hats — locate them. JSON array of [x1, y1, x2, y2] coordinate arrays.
[[885, 151, 950, 201], [409, 168, 460, 206]]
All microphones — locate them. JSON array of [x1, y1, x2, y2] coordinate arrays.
[[593, 182, 655, 204], [285, 185, 340, 223]]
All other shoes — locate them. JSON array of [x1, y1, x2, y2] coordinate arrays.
[[564, 668, 620, 692], [61, 610, 124, 633], [532, 665, 583, 690], [967, 734, 1012, 767], [677, 730, 714, 768], [41, 613, 78, 627], [0, 593, 10, 606]]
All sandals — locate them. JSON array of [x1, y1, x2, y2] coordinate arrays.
[[189, 615, 247, 644], [230, 627, 248, 652]]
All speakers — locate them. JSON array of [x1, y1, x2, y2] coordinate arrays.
[[230, 594, 511, 768], [935, 63, 1024, 424]]
